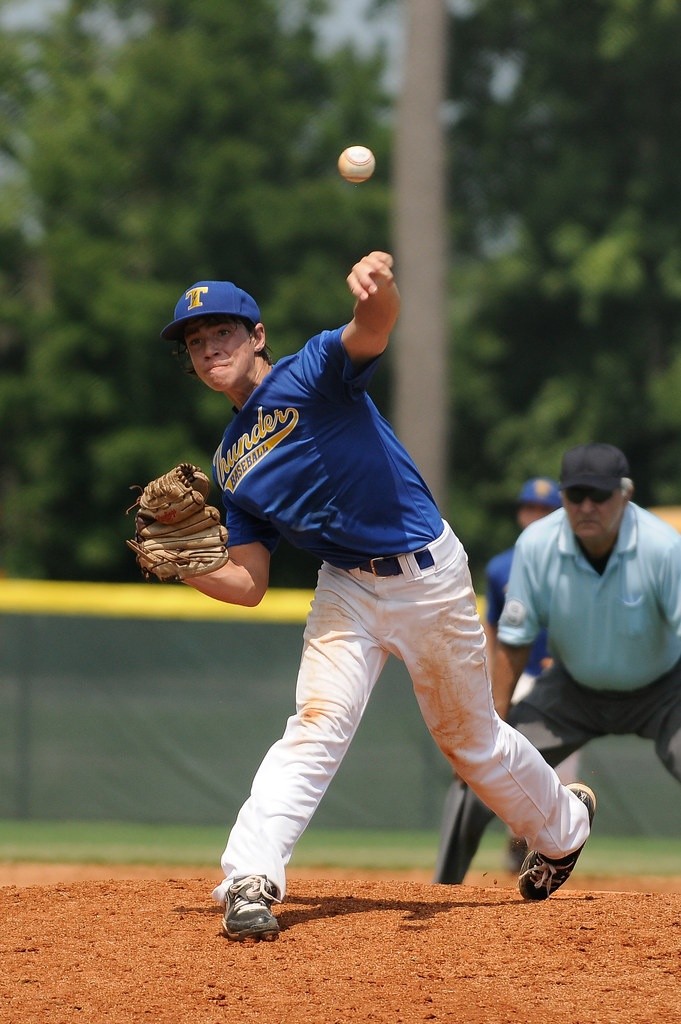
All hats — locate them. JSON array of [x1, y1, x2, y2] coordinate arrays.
[[558, 444, 629, 490], [161, 281, 260, 341], [518, 476, 563, 507]]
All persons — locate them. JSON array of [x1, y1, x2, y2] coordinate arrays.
[[479, 469, 582, 873], [430, 435, 681, 887], [136, 251, 598, 946]]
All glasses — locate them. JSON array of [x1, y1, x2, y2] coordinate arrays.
[[565, 486, 613, 504]]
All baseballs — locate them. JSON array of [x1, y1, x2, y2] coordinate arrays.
[[337, 145, 376, 183]]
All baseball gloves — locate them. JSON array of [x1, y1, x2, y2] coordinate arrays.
[[125, 462, 230, 584]]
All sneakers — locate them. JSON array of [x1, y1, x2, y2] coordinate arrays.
[[516, 783, 596, 900], [220, 874, 279, 939]]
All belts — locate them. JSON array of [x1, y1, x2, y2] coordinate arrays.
[[319, 549, 435, 578]]
